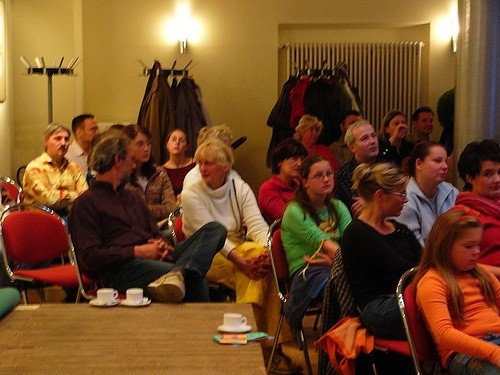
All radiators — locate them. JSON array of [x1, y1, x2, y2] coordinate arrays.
[[282, 41, 425, 134]]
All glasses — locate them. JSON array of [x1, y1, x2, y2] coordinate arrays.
[[307, 173, 334, 180]]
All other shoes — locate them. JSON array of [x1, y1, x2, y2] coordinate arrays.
[[147, 270, 185, 302]]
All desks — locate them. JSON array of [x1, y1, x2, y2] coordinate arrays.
[[0, 303, 266, 375]]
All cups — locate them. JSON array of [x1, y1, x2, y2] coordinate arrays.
[[97, 287, 118, 303], [223, 313, 247, 330], [126, 287, 144, 303]]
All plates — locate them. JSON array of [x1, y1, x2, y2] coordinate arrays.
[[89, 299, 120, 307], [218, 324, 253, 333], [121, 297, 152, 307]]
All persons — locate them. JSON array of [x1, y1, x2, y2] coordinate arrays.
[[66, 136, 228, 304], [65, 114, 99, 176], [294, 115, 338, 172], [411, 211, 500, 375], [335, 120, 378, 220], [258, 139, 308, 226], [122, 123, 176, 242], [405, 107, 435, 143], [281, 153, 351, 331], [22, 123, 89, 219], [0, 185, 13, 219], [330, 110, 362, 165], [340, 163, 424, 339], [437, 89, 455, 144], [444, 140, 455, 170], [452, 138, 500, 267], [163, 128, 196, 193], [179, 124, 304, 375], [394, 141, 460, 247], [375, 110, 416, 167]]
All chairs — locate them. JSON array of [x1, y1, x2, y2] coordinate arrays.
[[266, 217, 444, 375], [168, 206, 237, 303], [0, 176, 127, 304]]
